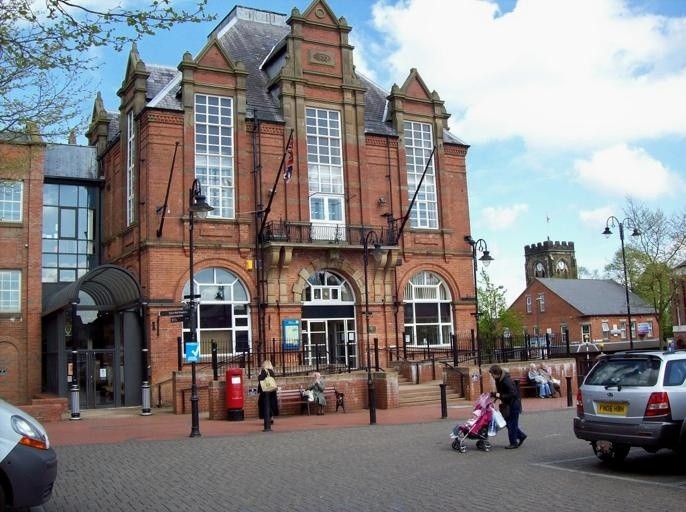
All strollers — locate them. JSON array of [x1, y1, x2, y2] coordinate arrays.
[[452, 391, 501, 453]]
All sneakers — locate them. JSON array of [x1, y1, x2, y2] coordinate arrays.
[[505, 444, 518, 449], [518, 436, 527, 446]]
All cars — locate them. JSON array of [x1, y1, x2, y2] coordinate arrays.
[[1, 396, 58, 506]]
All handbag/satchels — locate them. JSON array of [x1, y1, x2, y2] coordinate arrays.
[[488, 404, 506, 436], [300, 385, 314, 402], [260, 377, 278, 391]]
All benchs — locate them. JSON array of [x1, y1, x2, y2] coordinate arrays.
[[513, 376, 562, 398], [274, 385, 346, 415]]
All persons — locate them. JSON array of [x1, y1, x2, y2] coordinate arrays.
[[308, 371, 326, 415], [527, 362, 551, 397], [539, 361, 560, 397], [489, 364, 528, 449], [257, 360, 280, 425]]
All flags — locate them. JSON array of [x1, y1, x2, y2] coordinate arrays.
[[283, 134, 296, 184]]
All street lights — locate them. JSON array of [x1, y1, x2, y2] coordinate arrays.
[[362, 231, 382, 425], [601, 215, 643, 350], [188, 178, 216, 437], [471, 238, 496, 395]]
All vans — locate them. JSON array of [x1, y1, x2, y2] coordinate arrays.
[[571, 348, 686, 462]]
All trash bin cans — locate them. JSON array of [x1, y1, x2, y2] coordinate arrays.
[[224, 368, 244, 410]]
